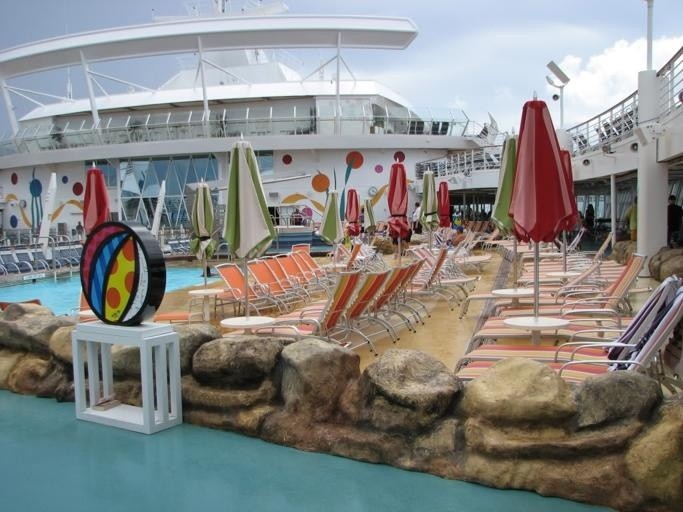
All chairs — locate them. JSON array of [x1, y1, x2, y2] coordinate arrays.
[[405, 120, 492, 138], [572, 102, 640, 153], [426, 151, 500, 179]]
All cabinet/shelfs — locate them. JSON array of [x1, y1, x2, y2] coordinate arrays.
[[70, 320, 184, 434]]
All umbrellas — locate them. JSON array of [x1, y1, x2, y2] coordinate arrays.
[[187, 179, 219, 289], [217, 135, 280, 316], [505, 88, 579, 321], [552, 142, 579, 274], [78, 166, 110, 239], [488, 130, 522, 291], [315, 158, 453, 272]]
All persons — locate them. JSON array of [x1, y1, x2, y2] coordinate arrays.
[[621, 195, 636, 242], [584, 202, 596, 241], [289, 207, 306, 225], [75, 220, 84, 239], [447, 225, 465, 246], [666, 194, 681, 246]]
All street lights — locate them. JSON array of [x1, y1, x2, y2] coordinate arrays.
[[544, 60, 569, 128]]
